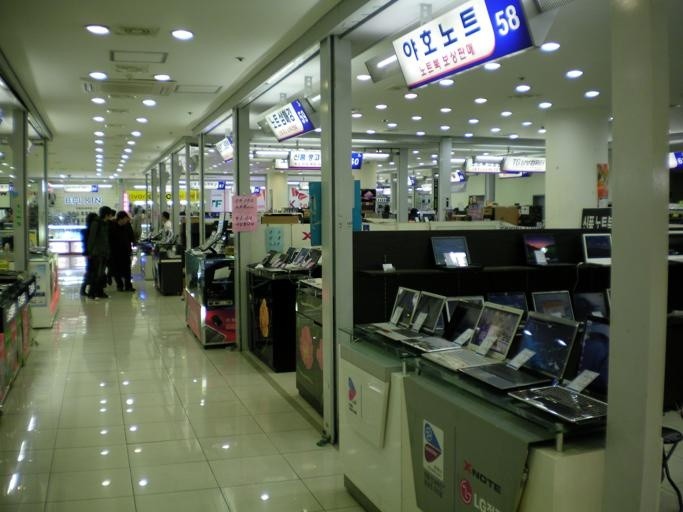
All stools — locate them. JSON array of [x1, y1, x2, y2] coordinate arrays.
[[656, 424, 682, 512]]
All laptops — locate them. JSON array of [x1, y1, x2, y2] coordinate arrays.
[[523, 233, 577, 268], [198, 212, 227, 252], [259, 247, 322, 273], [668, 230, 683, 264], [582, 233, 612, 266], [430, 235, 483, 271], [356, 286, 611, 425]]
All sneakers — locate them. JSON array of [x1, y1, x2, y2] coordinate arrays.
[[80, 275, 135, 300]]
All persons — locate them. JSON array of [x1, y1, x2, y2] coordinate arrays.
[[382, 205, 392, 218], [0, 207, 13, 251], [80, 205, 175, 299], [408, 207, 421, 221]]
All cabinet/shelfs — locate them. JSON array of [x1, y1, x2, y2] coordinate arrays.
[[181, 249, 238, 347]]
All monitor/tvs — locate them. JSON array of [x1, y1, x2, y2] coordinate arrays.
[[361, 189, 377, 202]]
